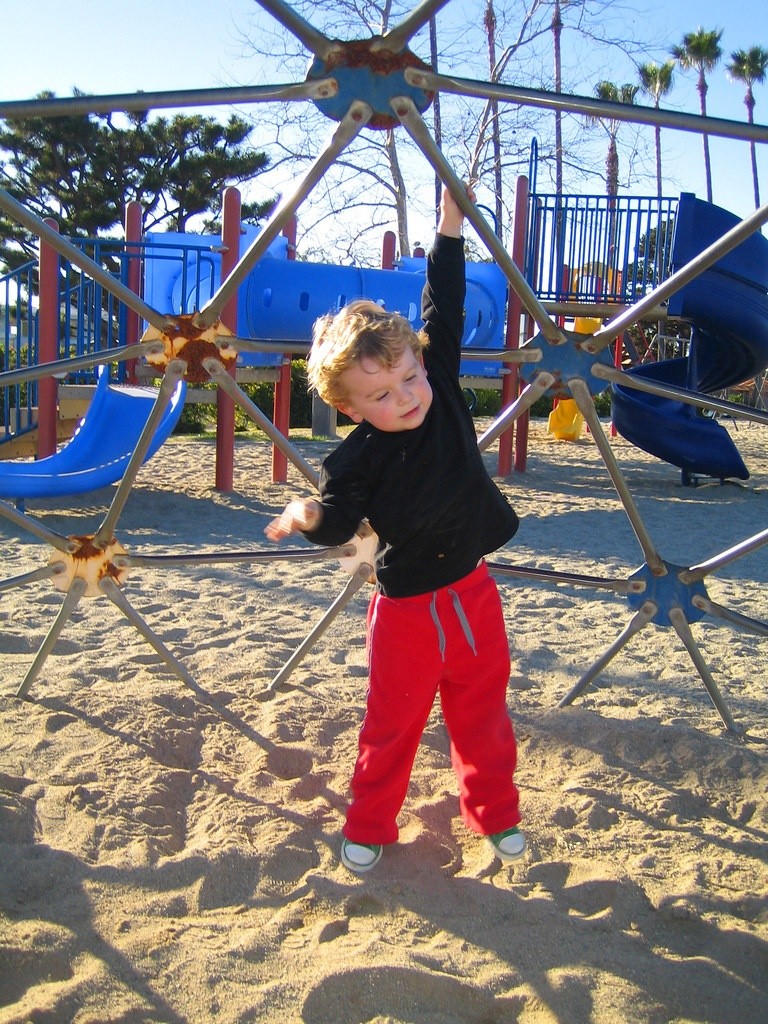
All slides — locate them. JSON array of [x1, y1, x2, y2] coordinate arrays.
[[612, 192, 767, 479], [1, 365, 186, 499]]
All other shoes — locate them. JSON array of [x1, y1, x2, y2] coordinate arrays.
[[342, 838, 384, 870], [486, 825, 529, 859]]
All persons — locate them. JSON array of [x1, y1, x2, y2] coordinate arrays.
[[263, 169, 536, 875]]
